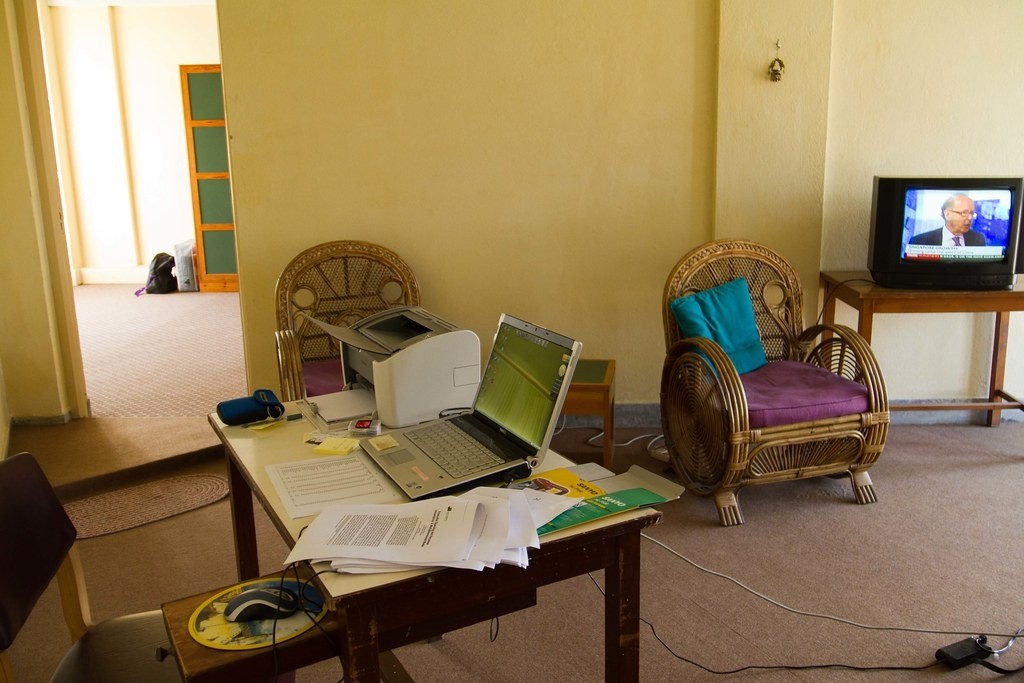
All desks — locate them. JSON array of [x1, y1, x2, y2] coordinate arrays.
[[206, 400, 665, 683], [819, 267, 1024, 429], [560, 358, 619, 467]]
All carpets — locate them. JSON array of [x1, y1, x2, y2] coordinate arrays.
[[59, 472, 230, 541]]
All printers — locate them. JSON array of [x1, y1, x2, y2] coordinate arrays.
[[296, 300, 481, 429]]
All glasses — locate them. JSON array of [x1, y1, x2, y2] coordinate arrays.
[[947, 209, 975, 217]]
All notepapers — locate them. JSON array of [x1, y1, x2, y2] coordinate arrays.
[[313, 436, 360, 456]]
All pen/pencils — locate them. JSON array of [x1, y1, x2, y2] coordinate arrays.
[[241, 413, 303, 428]]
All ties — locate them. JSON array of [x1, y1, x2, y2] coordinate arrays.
[[952, 237, 961, 246]]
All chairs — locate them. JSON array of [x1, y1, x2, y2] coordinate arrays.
[[275, 239, 422, 404], [658, 237, 889, 527], [0, 450, 185, 683]]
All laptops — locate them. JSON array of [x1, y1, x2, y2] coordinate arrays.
[[359, 314, 583, 501]]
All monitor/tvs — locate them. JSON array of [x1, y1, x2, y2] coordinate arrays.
[[867, 175, 1024, 290]]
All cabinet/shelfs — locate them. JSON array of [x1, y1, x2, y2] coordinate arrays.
[[179, 64, 239, 293]]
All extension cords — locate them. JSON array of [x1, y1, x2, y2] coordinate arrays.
[[650, 447, 670, 462]]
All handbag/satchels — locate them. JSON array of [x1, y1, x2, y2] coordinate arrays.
[[216, 389, 285, 426]]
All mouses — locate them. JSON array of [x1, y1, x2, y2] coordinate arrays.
[[223, 587, 298, 622]]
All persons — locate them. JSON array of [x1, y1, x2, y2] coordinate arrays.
[[905, 194, 986, 247]]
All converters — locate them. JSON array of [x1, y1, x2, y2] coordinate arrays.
[[936, 638, 993, 669]]
